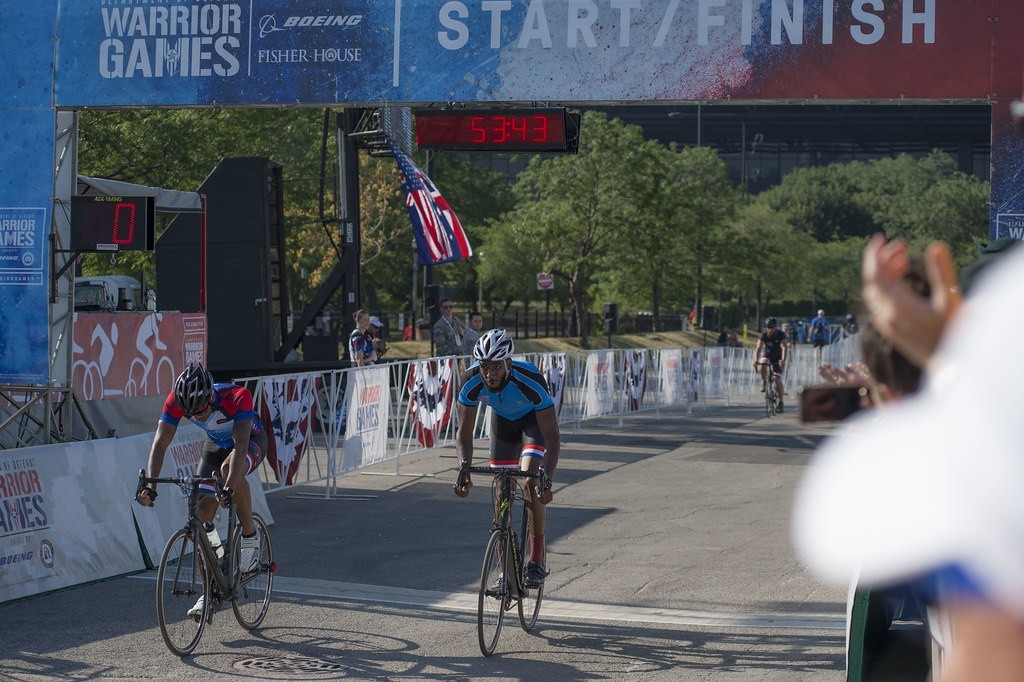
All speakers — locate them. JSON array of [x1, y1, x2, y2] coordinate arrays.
[[429, 284, 445, 323], [604, 302, 617, 331]]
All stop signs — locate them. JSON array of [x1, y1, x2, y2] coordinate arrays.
[[536, 272, 554, 289]]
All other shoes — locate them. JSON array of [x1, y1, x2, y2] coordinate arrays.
[[760, 385, 766, 392], [775, 404, 783, 413]]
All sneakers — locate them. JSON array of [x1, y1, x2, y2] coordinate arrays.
[[187, 594, 219, 617], [239, 526, 262, 572], [524, 561, 546, 589], [485, 577, 509, 597]]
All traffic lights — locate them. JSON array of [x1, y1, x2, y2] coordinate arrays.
[[70, 194, 147, 251]]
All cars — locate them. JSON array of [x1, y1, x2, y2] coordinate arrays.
[[403, 319, 422, 340]]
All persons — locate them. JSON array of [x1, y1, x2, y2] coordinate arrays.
[[349, 310, 377, 368], [453, 328, 560, 597], [717, 309, 858, 355], [432, 298, 465, 366], [462, 312, 482, 371], [137, 362, 268, 618], [403, 320, 420, 341], [364, 316, 385, 365], [754, 317, 788, 412], [793, 233, 1024, 682]]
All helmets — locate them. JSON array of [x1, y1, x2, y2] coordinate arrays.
[[766, 318, 777, 327], [473, 328, 515, 361], [175, 362, 214, 416], [846, 314, 857, 324]]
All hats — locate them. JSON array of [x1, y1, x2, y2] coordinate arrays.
[[369, 316, 384, 327]]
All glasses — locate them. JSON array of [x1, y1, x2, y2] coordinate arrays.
[[444, 305, 453, 310], [768, 326, 773, 329]]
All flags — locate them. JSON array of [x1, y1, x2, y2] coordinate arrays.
[[386, 140, 472, 266]]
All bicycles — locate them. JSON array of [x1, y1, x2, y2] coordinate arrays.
[[457, 457, 551, 657], [753, 361, 789, 418], [134, 468, 276, 656]]
